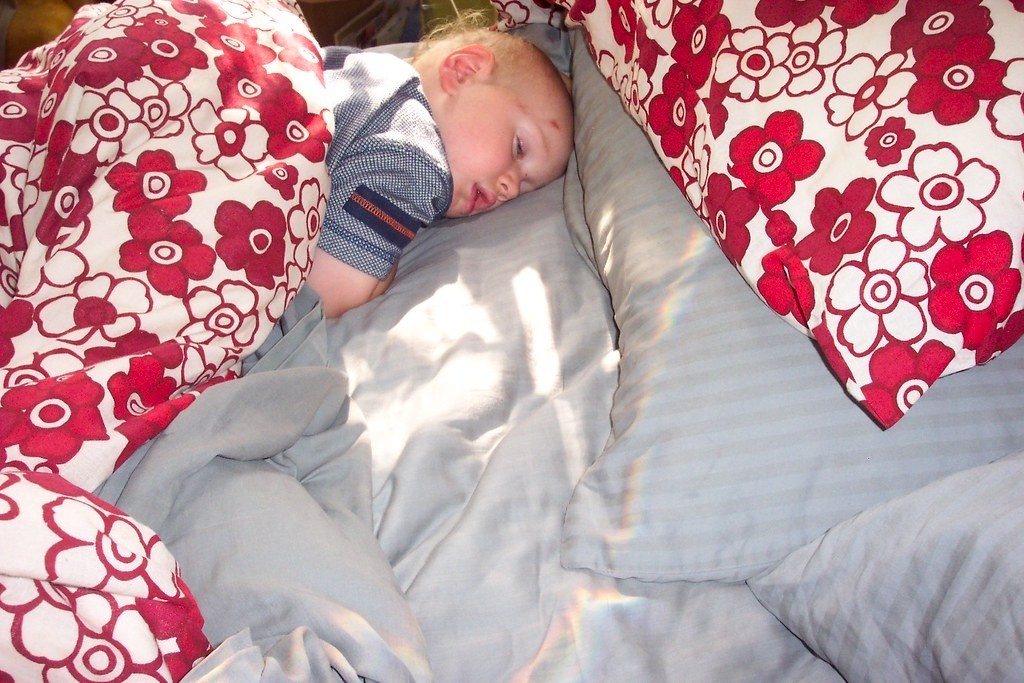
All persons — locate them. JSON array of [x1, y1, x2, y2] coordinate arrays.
[[301, 24, 576, 316]]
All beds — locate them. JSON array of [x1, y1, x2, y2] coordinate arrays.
[[0, 0, 1024, 683]]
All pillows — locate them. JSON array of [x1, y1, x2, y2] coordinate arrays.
[[561, 27, 1023, 585], [548, 0, 1024, 431], [745, 448, 1024, 683]]
